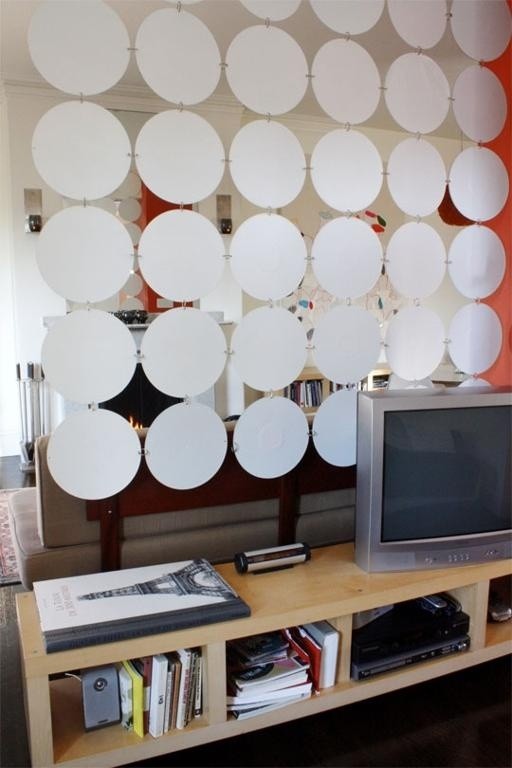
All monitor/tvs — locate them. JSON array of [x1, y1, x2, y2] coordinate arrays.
[[354, 386, 512, 573]]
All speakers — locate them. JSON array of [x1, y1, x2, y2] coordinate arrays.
[[82, 666, 122, 733]]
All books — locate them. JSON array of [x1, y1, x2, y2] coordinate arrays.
[[117, 620, 341, 738], [33, 559, 250, 654], [284, 377, 387, 409]]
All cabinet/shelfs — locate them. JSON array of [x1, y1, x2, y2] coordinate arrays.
[[12, 539, 512, 768], [262, 363, 392, 414]]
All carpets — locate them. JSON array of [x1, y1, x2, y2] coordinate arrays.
[[0, 486, 24, 586]]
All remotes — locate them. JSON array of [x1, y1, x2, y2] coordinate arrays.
[[422, 595, 447, 608]]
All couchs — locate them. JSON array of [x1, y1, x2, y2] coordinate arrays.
[[6, 409, 355, 594]]
